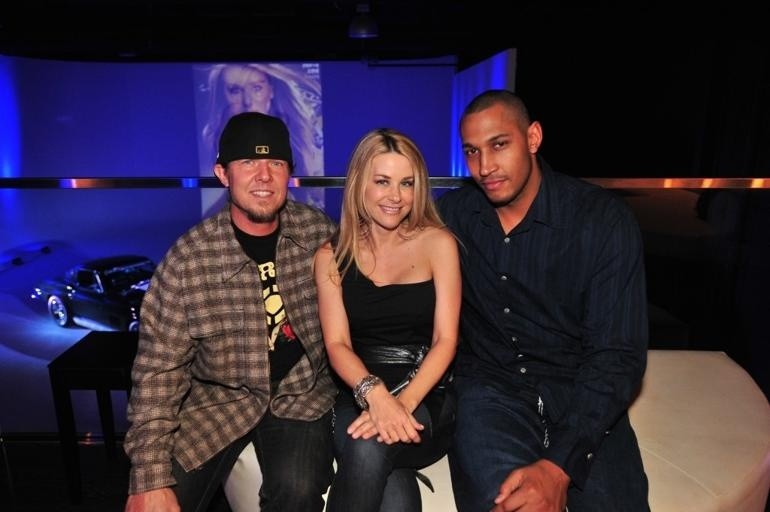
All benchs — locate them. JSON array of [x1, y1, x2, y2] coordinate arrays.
[[223, 350, 770, 512]]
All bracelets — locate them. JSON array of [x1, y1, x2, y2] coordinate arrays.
[[352, 373, 385, 409]]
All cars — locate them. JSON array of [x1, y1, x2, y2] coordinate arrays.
[[30, 254, 157, 330]]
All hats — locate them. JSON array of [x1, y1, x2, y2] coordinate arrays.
[[219, 112, 293, 168]]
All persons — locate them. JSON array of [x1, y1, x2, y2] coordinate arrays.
[[306, 130, 465, 511], [120, 109, 337, 510], [192, 60, 324, 219], [432, 88, 660, 510]]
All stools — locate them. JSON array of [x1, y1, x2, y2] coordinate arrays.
[[46, 331, 139, 504]]
